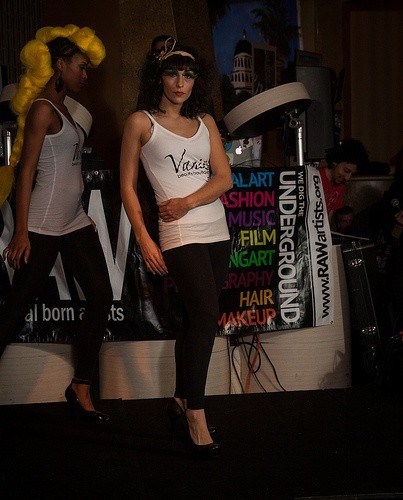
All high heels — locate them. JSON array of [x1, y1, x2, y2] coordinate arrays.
[[166, 399, 224, 454], [65, 384, 110, 423]]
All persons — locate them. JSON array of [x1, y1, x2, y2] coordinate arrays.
[[152, 35, 178, 52], [318, 140, 403, 343], [1, 25, 113, 426], [119, 38, 236, 459]]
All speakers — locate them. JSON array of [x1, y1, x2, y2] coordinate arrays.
[[282, 65, 334, 161]]
